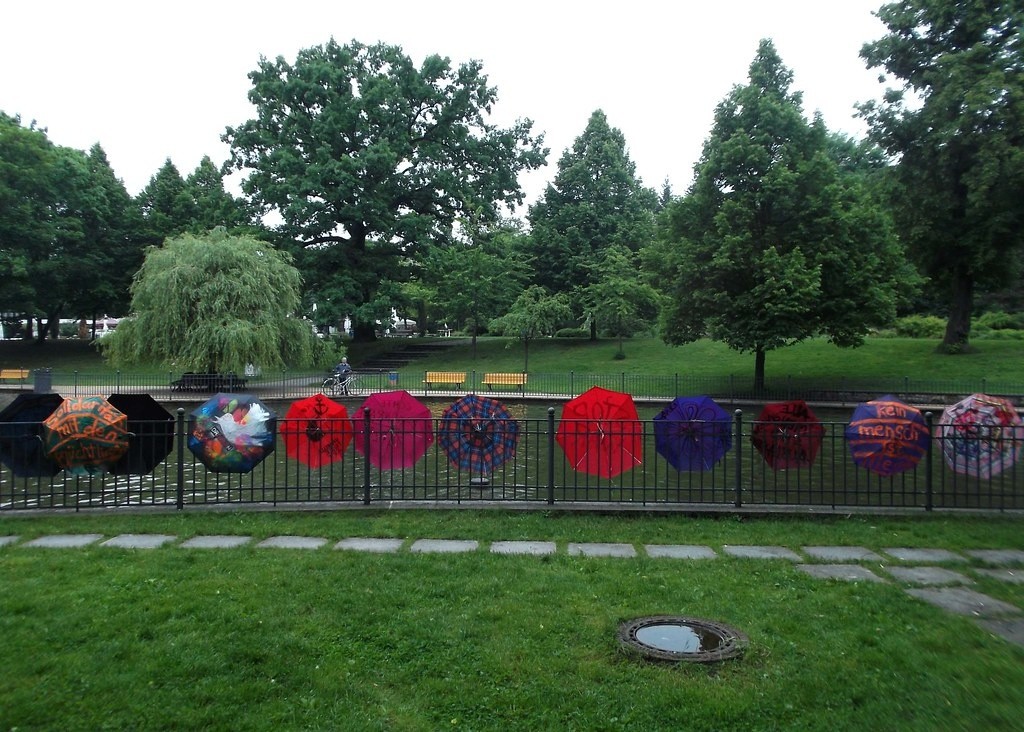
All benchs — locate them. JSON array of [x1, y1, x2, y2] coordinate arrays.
[[422, 372, 466, 390], [171, 374, 248, 392], [0, 369, 29, 383], [482, 373, 527, 391]]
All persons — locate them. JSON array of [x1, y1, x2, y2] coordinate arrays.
[[333, 356, 352, 396]]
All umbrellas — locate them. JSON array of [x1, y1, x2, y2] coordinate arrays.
[[351, 390, 434, 469], [437, 394, 521, 476], [186, 391, 278, 473], [555, 385, 643, 479], [845, 394, 931, 477], [106, 394, 175, 476], [43, 394, 128, 476], [0, 394, 64, 477], [750, 400, 827, 469], [937, 393, 1024, 479], [280, 394, 354, 468], [652, 394, 733, 472]]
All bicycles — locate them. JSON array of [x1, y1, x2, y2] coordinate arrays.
[[322, 371, 364, 397]]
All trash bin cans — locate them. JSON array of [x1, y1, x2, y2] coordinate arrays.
[[33, 368, 52, 394]]
